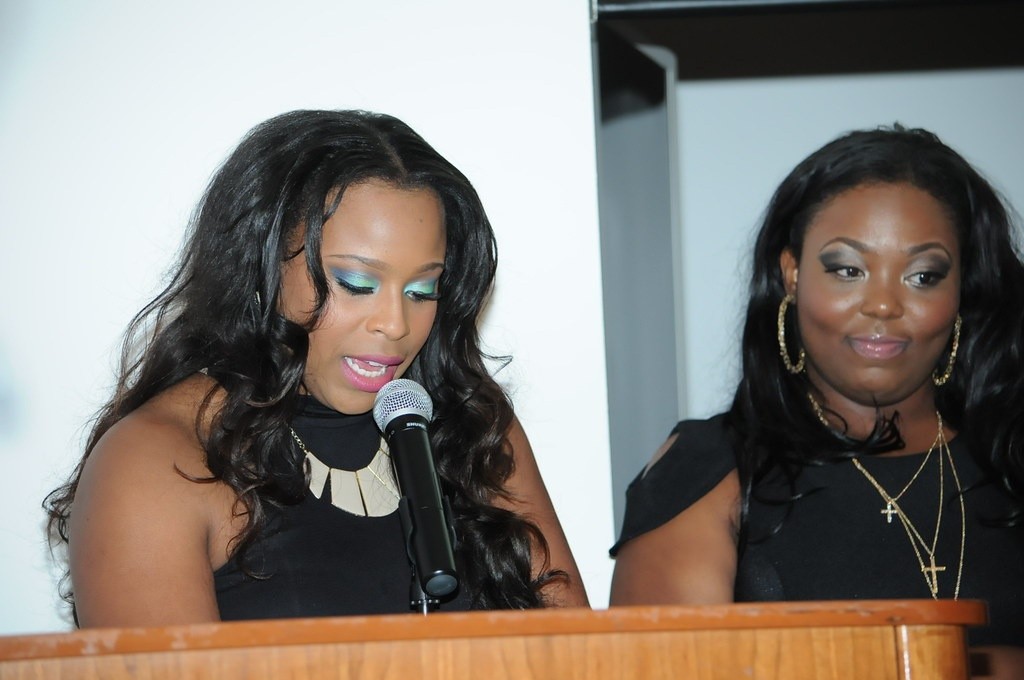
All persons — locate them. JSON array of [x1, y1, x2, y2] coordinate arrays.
[[43, 109, 591, 631], [610, 123, 1024, 680]]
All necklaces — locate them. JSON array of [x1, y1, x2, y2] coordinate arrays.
[[292, 428, 405, 518], [808, 390, 966, 600]]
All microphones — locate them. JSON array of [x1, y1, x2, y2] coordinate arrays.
[[372, 378, 459, 596]]
